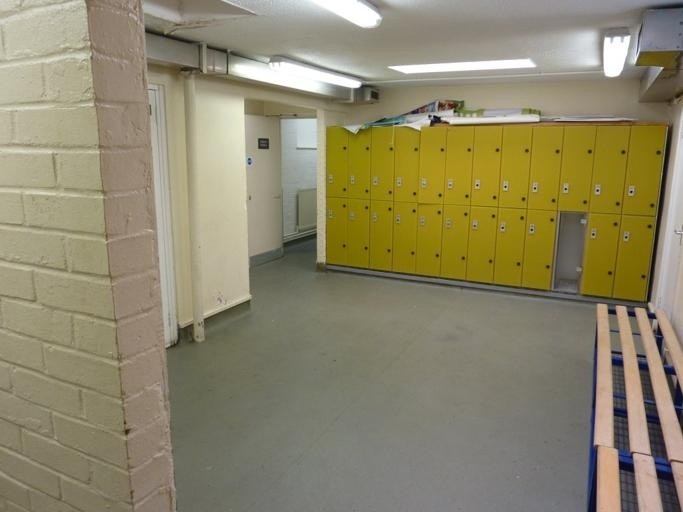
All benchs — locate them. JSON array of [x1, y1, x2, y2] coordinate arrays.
[[587, 303, 683, 511]]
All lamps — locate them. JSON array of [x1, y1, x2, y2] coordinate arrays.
[[601, 28, 631, 79], [309, 0, 380, 30], [269, 56, 362, 91]]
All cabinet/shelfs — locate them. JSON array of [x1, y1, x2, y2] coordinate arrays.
[[325, 125, 670, 303]]
